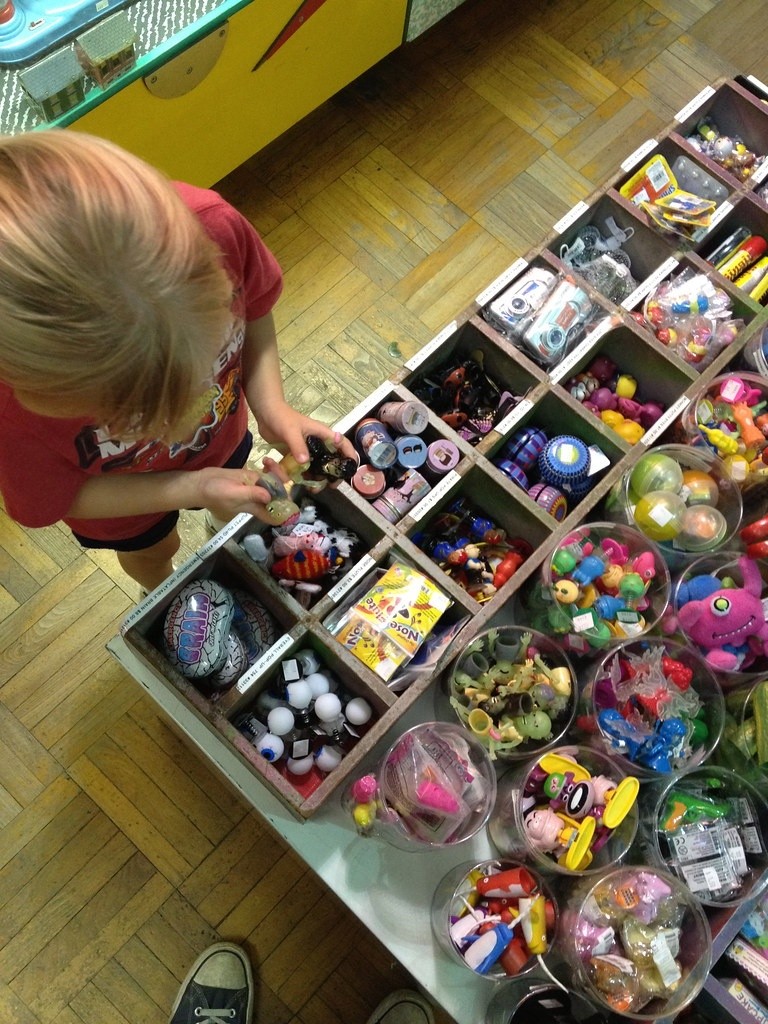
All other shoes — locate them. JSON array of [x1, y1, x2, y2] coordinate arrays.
[[204, 512, 219, 534], [141, 564, 178, 596]]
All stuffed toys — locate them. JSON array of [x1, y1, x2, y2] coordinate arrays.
[[677, 555, 768, 672], [274, 520, 359, 593]]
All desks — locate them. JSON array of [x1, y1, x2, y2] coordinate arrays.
[[103, 565, 534, 1024]]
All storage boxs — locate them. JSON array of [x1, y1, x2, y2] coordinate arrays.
[[120, 76, 768, 816]]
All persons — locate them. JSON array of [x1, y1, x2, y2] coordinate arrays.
[[167, 941, 435, 1024], [0, 131, 358, 598], [450, 628, 572, 760], [465, 544, 485, 583]]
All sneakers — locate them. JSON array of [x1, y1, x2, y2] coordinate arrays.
[[168, 942, 254, 1024], [365, 990, 435, 1024]]
